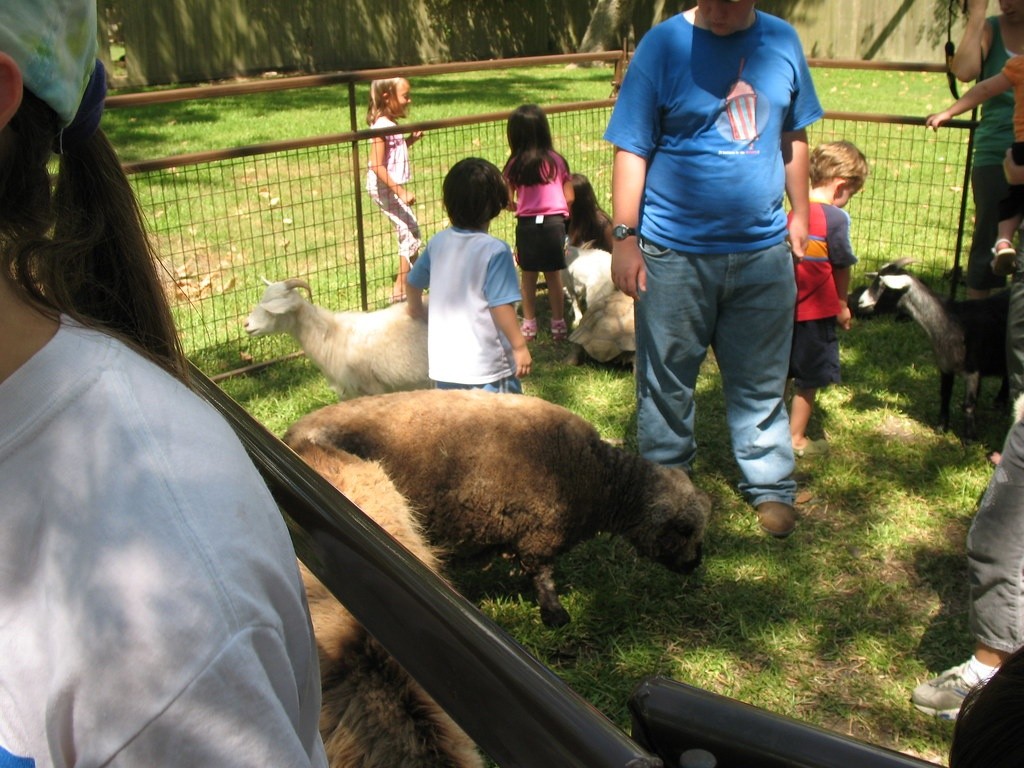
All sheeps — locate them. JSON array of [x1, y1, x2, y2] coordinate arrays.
[[561, 239, 623, 329], [855, 255, 1011, 446], [267, 385, 710, 768], [241, 278, 435, 398]]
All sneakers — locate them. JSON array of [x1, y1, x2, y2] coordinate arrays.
[[552, 322, 568, 340], [912, 661, 983, 720], [757, 502, 795, 537], [793, 436, 828, 454], [521, 324, 537, 341]]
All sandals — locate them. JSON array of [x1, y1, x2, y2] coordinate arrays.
[[991, 239, 1016, 277]]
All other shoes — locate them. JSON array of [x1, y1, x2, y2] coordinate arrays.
[[390, 294, 425, 303]]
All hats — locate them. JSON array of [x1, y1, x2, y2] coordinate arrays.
[[0, 0, 99, 129]]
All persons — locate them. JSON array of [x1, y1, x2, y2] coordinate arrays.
[[565, 173, 613, 255], [925, 53, 1024, 277], [602, 0, 824, 537], [984, 148, 1024, 469], [950, 0, 1024, 301], [912, 414, 1024, 720], [501, 104, 575, 342], [783, 139, 869, 462], [0, 0, 330, 768], [404, 157, 533, 394], [948, 645, 1024, 768], [365, 77, 424, 303]]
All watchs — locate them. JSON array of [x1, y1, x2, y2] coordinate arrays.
[[611, 223, 638, 241]]
